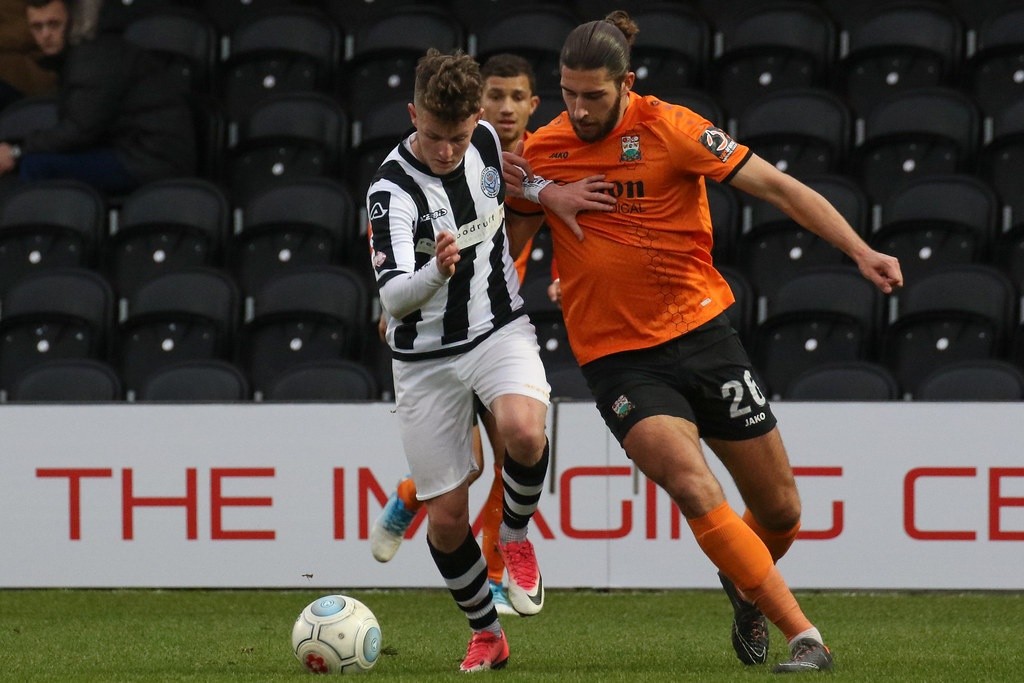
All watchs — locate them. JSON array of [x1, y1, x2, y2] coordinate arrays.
[[10, 143, 22, 165]]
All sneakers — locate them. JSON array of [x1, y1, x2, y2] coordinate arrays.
[[717, 568, 769, 666], [486, 581, 519, 617], [368, 473, 418, 563], [460, 626, 509, 674], [774, 636, 831, 674], [498, 538, 546, 615]]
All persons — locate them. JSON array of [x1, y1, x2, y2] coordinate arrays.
[[0, 0, 194, 194], [366, 54, 623, 674], [496, 13, 905, 672], [369, 55, 547, 619]]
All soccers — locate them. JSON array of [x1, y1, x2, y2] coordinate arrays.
[[290, 593, 384, 675]]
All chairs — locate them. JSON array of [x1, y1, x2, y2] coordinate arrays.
[[0, 0, 1024, 404]]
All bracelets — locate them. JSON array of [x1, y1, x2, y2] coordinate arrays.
[[520, 176, 554, 206]]
